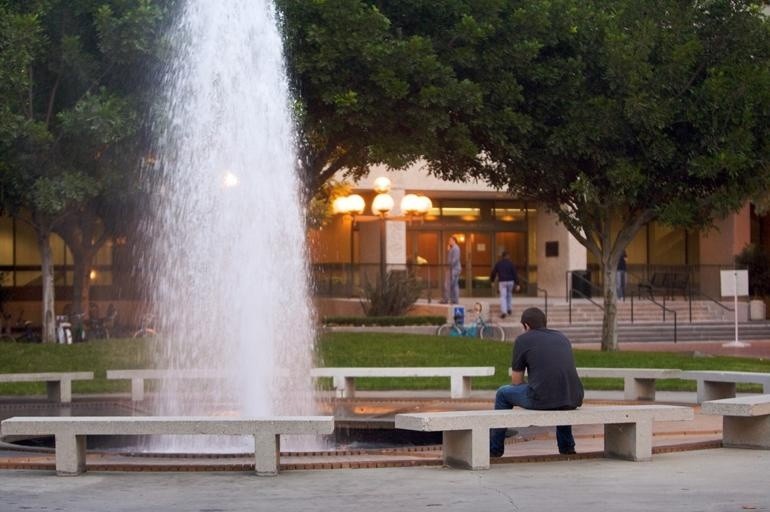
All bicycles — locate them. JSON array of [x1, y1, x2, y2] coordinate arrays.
[[0, 303, 157, 346], [437, 303, 505, 343]]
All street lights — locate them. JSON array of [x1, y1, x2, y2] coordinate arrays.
[[369, 176, 393, 277], [333, 193, 366, 297], [401, 192, 433, 277]]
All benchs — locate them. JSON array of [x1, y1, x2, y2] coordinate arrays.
[[105, 365, 217, 401], [508, 364, 683, 402], [392, 404, 696, 472], [0, 413, 338, 482], [698, 392, 770, 452], [309, 364, 497, 404], [0, 367, 94, 407], [679, 368, 770, 407]]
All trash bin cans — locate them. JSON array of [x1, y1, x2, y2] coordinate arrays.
[[571, 270, 592, 299]]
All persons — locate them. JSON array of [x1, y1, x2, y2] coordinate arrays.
[[616, 249, 628, 303], [491, 251, 520, 318], [438, 236, 462, 304], [100, 303, 118, 339], [489, 308, 585, 454], [77, 300, 101, 338]]
